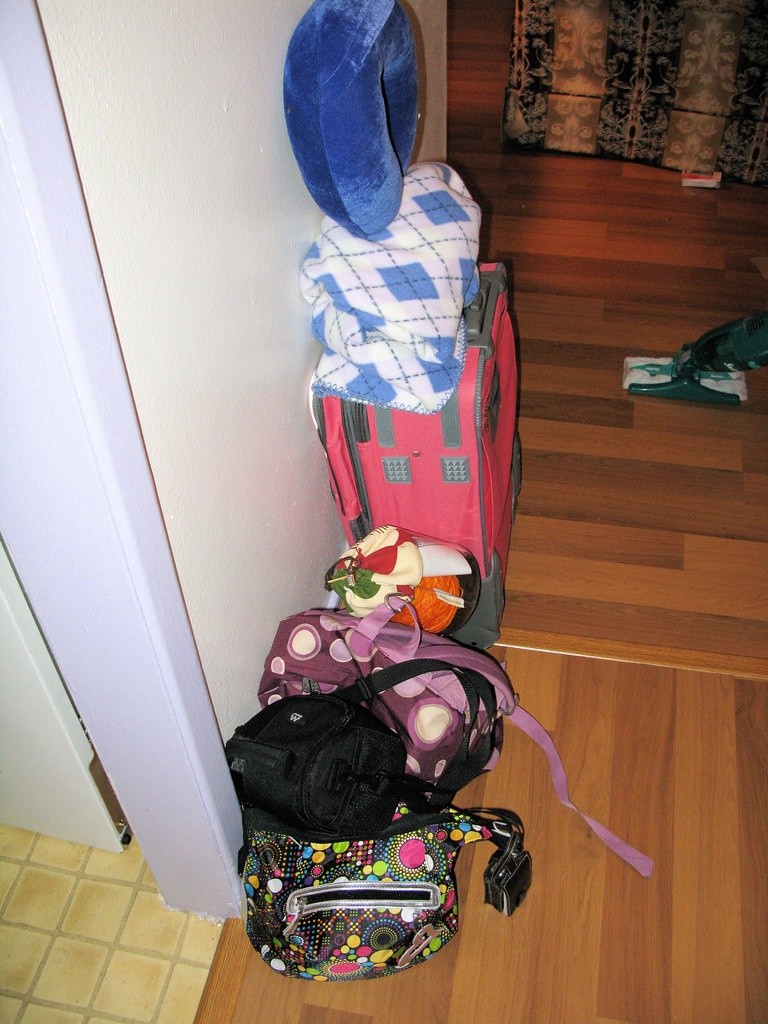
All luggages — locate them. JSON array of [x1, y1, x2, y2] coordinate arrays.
[[310, 260, 522, 652]]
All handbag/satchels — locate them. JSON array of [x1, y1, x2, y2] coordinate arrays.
[[220, 593, 532, 982]]
